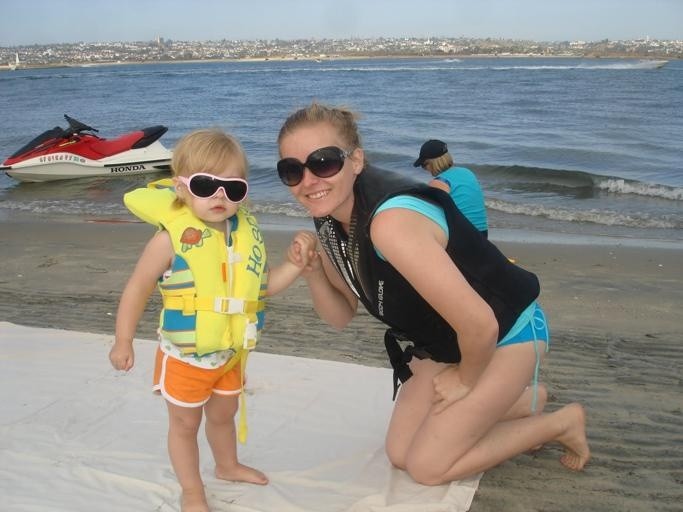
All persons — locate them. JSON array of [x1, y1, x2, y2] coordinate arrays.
[[411, 138, 488, 243], [106, 127, 316, 510], [276, 104, 591, 485]]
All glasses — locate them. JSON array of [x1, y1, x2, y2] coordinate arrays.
[[276, 145, 356, 187], [177, 172, 249, 204]]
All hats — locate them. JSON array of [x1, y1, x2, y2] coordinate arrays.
[[413, 139, 448, 167]]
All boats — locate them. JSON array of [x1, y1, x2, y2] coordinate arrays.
[[0, 114, 173, 183]]
